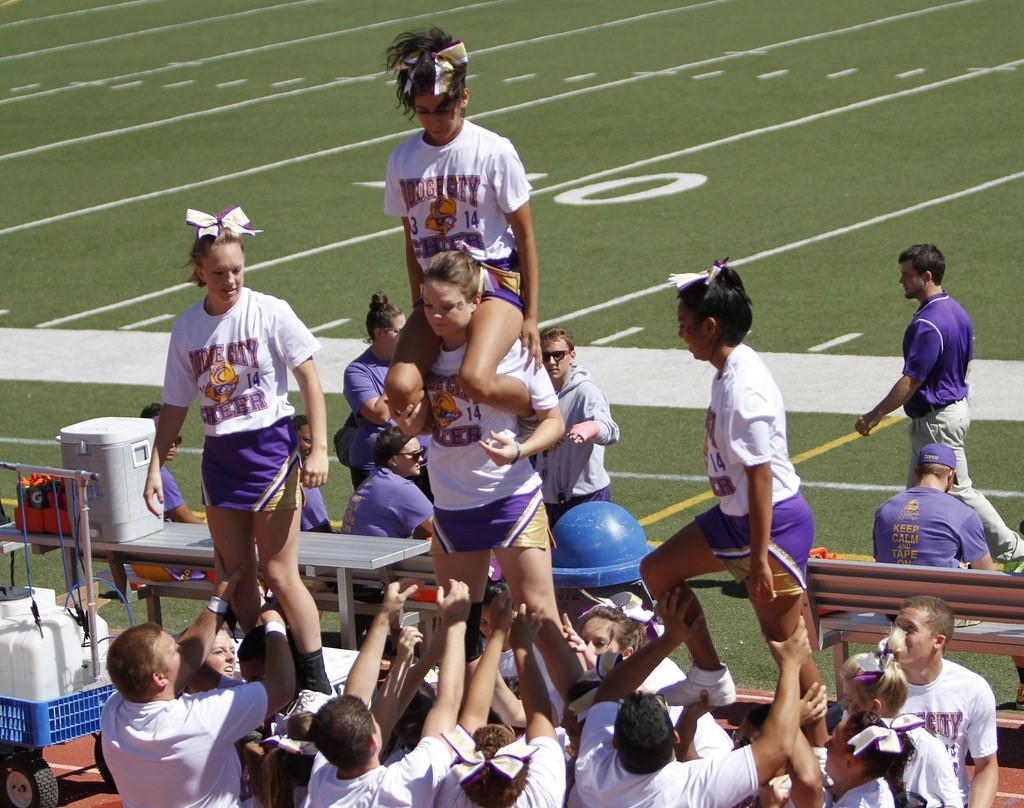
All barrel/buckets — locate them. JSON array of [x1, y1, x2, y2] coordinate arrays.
[[55, 416, 164, 542], [0, 581, 109, 699]]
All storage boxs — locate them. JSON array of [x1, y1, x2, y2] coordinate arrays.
[[0, 682, 115, 748]]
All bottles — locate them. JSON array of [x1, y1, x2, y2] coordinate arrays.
[[13, 465, 75, 535]]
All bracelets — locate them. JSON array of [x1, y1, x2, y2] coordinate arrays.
[[511, 441, 520, 464], [265, 621, 286, 635], [207, 596, 228, 613]]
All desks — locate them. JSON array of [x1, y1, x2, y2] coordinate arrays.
[[0, 517, 431, 653]]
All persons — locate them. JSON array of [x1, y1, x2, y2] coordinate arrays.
[[384, 25, 542, 436], [99, 208, 1000, 808], [855, 243, 1024, 564], [873, 442, 1024, 709]]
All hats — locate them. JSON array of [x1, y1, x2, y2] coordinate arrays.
[[918, 443, 959, 485]]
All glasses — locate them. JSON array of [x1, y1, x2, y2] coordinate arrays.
[[397, 446, 425, 463], [175, 436, 182, 444], [540, 352, 572, 361]]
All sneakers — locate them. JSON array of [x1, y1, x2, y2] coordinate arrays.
[[284, 684, 339, 714], [655, 663, 737, 708], [779, 746, 834, 789]]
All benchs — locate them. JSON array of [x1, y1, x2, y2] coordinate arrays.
[[800, 558, 1024, 725], [35, 526, 439, 663]]
